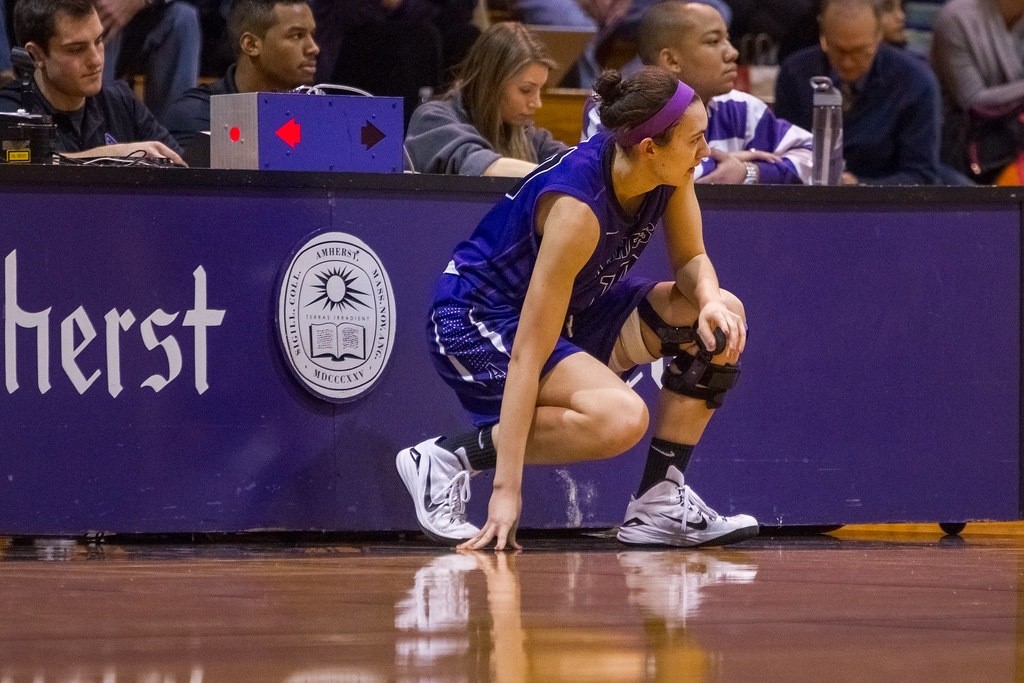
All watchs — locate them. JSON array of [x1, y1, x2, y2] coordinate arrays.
[[743, 161, 757, 185]]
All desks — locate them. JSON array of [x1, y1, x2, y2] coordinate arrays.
[[0, 162, 1024, 535]]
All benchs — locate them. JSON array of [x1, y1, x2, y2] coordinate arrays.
[[489, 23, 594, 147]]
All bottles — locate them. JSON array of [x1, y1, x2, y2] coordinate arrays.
[[809, 76, 843, 185]]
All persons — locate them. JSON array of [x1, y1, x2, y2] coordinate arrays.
[[0, 0, 1024, 191], [396, 66, 759, 551]]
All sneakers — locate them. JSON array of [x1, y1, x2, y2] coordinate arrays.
[[615, 465, 760, 548], [394, 435, 483, 547]]
